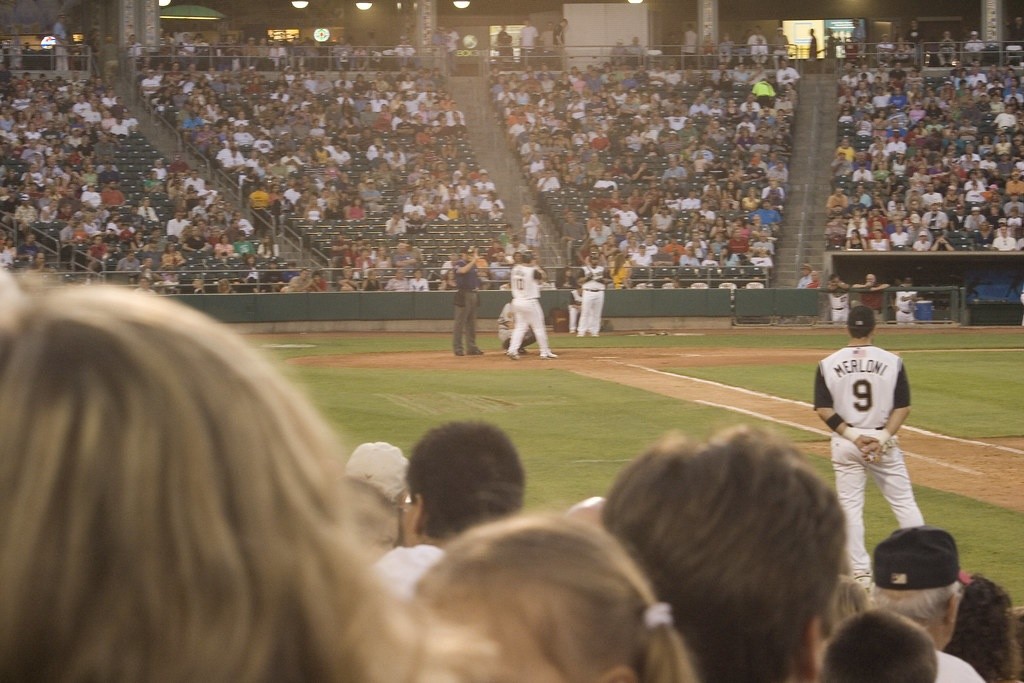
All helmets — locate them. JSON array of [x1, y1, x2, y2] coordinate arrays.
[[522, 250, 537, 263]]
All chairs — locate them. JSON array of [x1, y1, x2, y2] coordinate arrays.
[[34, 77, 992, 293]]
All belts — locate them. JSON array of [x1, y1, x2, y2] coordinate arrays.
[[848, 424, 884, 430], [585, 289, 601, 292]]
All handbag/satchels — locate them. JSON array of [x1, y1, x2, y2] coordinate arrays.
[[453, 287, 466, 307]]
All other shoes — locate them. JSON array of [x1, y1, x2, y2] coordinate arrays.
[[540, 352, 558, 359], [507, 352, 521, 362], [570, 330, 575, 334], [592, 332, 599, 337], [519, 348, 526, 353], [468, 349, 484, 355], [456, 349, 465, 357], [576, 331, 584, 338]]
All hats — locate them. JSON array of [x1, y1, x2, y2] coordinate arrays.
[[919, 231, 928, 237], [847, 306, 874, 327], [462, 246, 474, 254], [872, 526, 973, 591], [970, 206, 980, 212], [346, 442, 410, 502]]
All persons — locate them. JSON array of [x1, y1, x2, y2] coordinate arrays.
[[506, 248, 559, 361], [944, 574, 1024, 682], [601, 422, 847, 681], [573, 252, 613, 338], [410, 508, 707, 683], [812, 305, 925, 592], [2, 9, 1022, 681], [868, 526, 987, 682], [0, 275, 504, 683], [451, 246, 486, 357], [820, 607, 940, 682]]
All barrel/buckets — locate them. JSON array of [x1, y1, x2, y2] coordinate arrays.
[[916, 301, 934, 320]]
